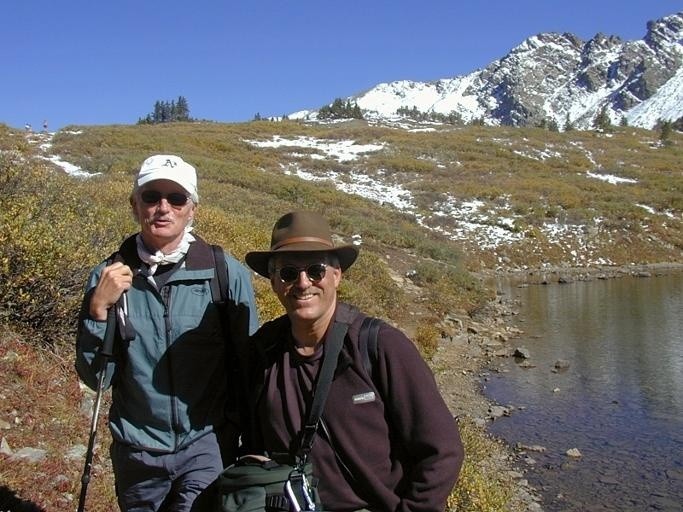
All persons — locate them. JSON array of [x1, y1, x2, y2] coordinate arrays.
[[226, 209, 465, 511], [75, 152, 259, 512]]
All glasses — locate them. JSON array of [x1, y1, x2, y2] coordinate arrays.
[[274, 262, 331, 284], [141, 190, 189, 206]]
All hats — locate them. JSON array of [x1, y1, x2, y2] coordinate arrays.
[[245, 211, 359, 280], [134, 155, 198, 200]]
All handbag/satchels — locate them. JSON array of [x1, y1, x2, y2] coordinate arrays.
[[212, 454, 322, 512]]
